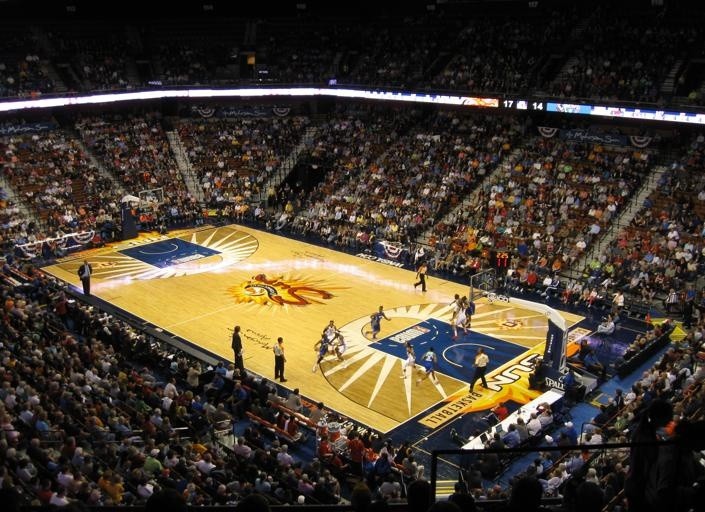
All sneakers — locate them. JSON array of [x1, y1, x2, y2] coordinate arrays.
[[400, 365, 442, 387], [312, 350, 347, 373], [468, 383, 488, 392], [414, 283, 427, 293], [451, 319, 471, 341]]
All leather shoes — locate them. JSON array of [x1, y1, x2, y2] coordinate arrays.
[[275, 375, 288, 382]]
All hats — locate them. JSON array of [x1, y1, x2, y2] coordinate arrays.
[[564, 421, 574, 428], [545, 435, 554, 444], [667, 223, 677, 230], [298, 495, 306, 505], [150, 448, 161, 456]]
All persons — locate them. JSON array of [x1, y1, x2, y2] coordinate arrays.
[[473, 290, 705, 512], [400, 341, 418, 379], [469, 347, 490, 393], [366, 306, 391, 339], [323, 320, 338, 355], [416, 346, 440, 387], [312, 334, 333, 373], [232, 326, 244, 371], [1, 264, 152, 362], [413, 261, 428, 292], [241, 0, 426, 251], [330, 329, 346, 362], [2, 1, 246, 264], [1, 354, 477, 512], [273, 337, 288, 382], [78, 260, 92, 294], [430, 2, 705, 309], [449, 294, 475, 339]]
